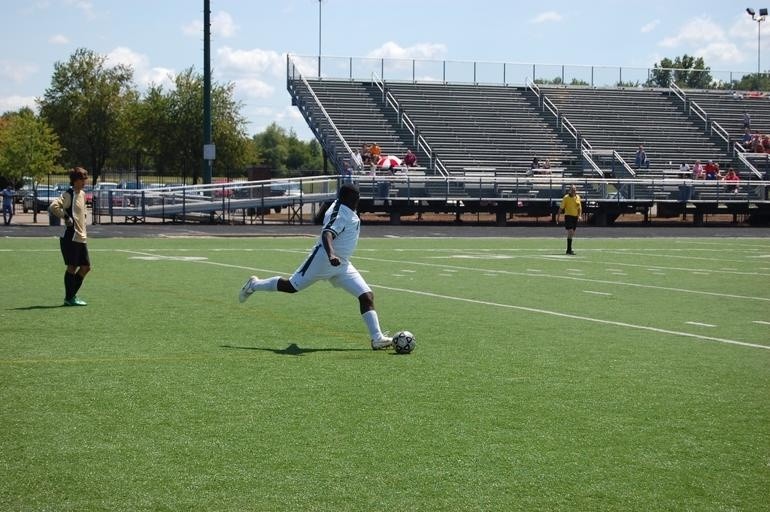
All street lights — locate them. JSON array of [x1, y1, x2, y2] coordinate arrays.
[[746, 8, 768, 72]]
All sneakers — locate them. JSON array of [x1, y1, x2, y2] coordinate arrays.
[[64, 295, 87, 306], [239, 276, 259, 303], [371, 334, 393, 350]]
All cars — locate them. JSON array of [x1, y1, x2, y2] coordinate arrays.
[[212, 178, 304, 196], [17, 176, 205, 213]]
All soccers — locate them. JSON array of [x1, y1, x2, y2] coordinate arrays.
[[392, 331, 416, 353]]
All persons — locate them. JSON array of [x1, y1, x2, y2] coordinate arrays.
[[92, 191, 99, 225], [721, 167, 741, 194], [543, 158, 552, 174], [678, 161, 693, 179], [704, 159, 716, 179], [237, 181, 394, 352], [531, 157, 542, 174], [713, 162, 722, 180], [48, 165, 93, 307], [0, 186, 18, 225], [122, 196, 130, 224], [634, 144, 651, 172], [556, 183, 583, 255], [133, 191, 140, 223], [693, 159, 706, 180], [730, 88, 770, 155], [340, 140, 416, 197]]
